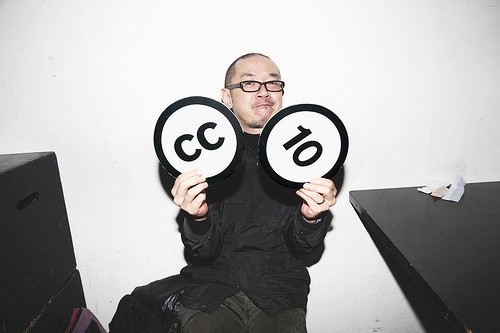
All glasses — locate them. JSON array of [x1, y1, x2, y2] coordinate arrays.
[[226, 79, 285, 91]]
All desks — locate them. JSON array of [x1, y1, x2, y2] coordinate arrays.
[[349, 181, 500, 333]]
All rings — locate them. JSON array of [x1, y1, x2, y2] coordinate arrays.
[[317, 200, 324, 204]]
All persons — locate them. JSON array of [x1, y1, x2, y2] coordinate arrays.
[[170, 52, 337, 333]]
